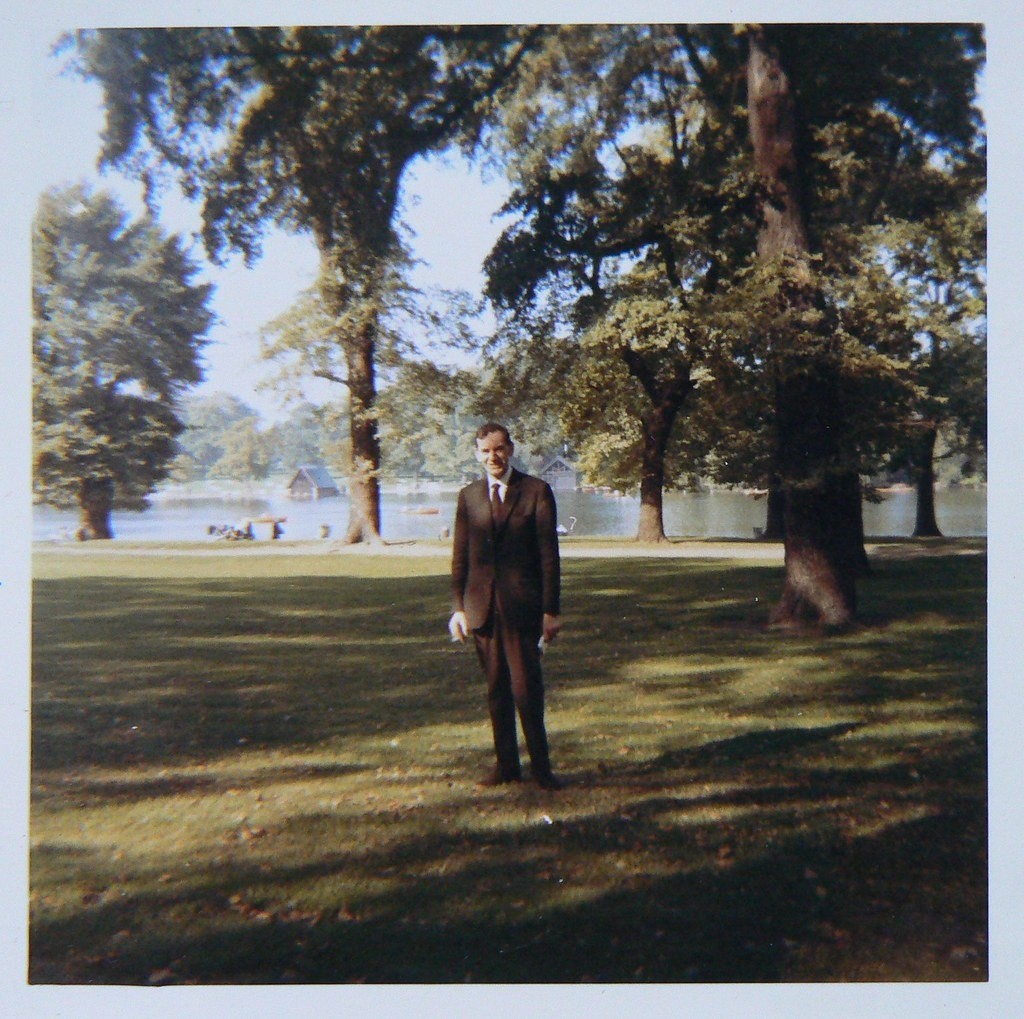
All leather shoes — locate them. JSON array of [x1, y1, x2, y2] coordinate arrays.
[[533, 770, 565, 790], [475, 768, 522, 786]]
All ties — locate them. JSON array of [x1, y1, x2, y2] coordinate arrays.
[[490, 483, 503, 526]]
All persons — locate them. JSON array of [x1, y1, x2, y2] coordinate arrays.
[[446, 422, 565, 792]]
